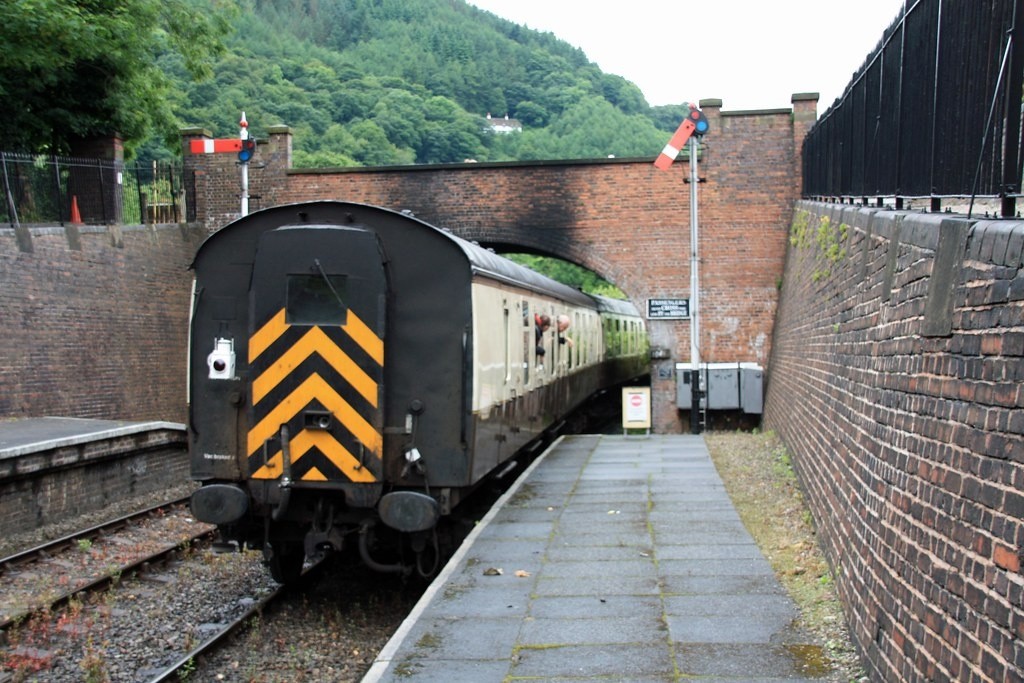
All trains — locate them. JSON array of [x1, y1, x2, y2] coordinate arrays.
[[183, 199, 649, 609]]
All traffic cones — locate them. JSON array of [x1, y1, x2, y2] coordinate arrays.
[[70, 194, 84, 225]]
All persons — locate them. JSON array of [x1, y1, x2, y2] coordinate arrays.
[[558, 315, 574, 349], [535, 313, 550, 364]]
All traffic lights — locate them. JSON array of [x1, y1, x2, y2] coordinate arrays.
[[233, 135, 256, 164], [687, 107, 708, 136]]
